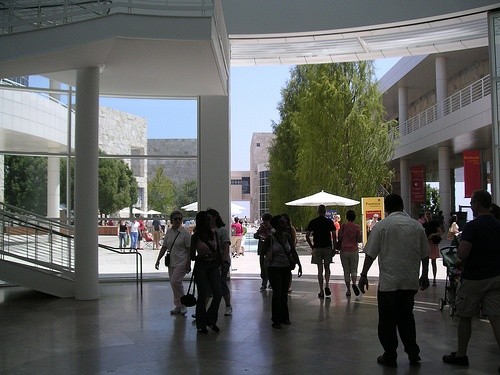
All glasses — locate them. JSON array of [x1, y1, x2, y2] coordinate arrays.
[[489, 210, 496, 214], [470, 200, 481, 205], [171, 216, 182, 221]]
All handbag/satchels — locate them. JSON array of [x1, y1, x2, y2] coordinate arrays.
[[165, 251, 170, 266], [431, 235, 442, 244], [258, 236, 266, 255], [287, 249, 297, 270], [180, 294, 197, 307], [335, 224, 345, 250], [213, 250, 221, 268]]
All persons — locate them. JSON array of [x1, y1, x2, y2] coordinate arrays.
[[418, 210, 445, 286], [152, 216, 162, 250], [190, 211, 221, 333], [368, 214, 379, 231], [443, 190, 500, 367], [307, 205, 337, 297], [253, 213, 297, 292], [155, 210, 191, 315], [191, 208, 233, 319], [230, 216, 247, 257], [331, 210, 362, 296], [264, 215, 302, 330], [118, 215, 144, 253], [358, 193, 430, 367]]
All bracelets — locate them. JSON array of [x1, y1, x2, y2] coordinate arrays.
[[360, 273, 367, 279]]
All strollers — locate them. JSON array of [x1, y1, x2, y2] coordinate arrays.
[[438, 246, 483, 319]]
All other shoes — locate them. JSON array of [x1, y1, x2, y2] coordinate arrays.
[[268, 285, 272, 289], [352, 285, 360, 296], [377, 356, 398, 368], [325, 288, 331, 295], [272, 323, 281, 329], [318, 292, 324, 298], [282, 320, 291, 325], [260, 286, 266, 290], [346, 291, 351, 296], [408, 353, 421, 362], [442, 352, 468, 366], [170, 305, 232, 335], [433, 279, 437, 286], [288, 288, 292, 292]]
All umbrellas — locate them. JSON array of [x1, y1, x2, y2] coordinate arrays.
[[146, 210, 162, 217], [285, 191, 360, 206], [120, 208, 145, 214], [181, 198, 246, 212]]
